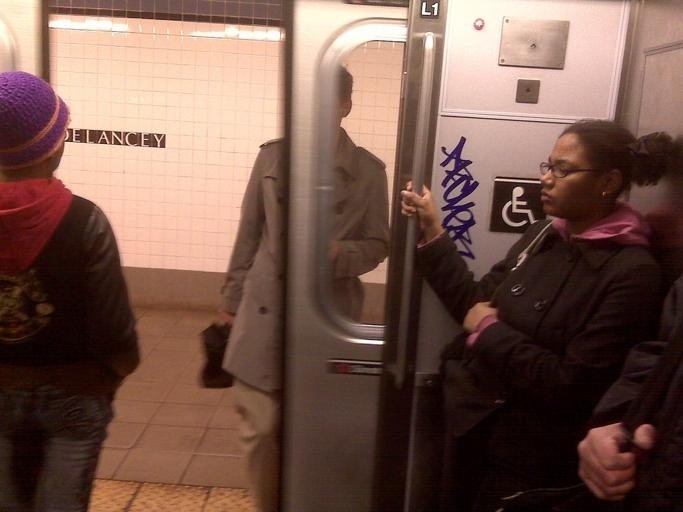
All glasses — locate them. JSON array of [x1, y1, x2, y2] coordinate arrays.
[[540, 161, 604, 178]]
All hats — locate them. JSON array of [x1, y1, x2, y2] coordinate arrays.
[[0, 70, 70, 171]]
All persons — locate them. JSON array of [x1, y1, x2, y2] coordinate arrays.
[[403, 120, 674, 511], [213, 64, 390, 512], [501, 276, 682, 511], [0, 70, 141, 512]]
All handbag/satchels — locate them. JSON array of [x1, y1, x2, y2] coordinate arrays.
[[202, 321, 234, 389]]
